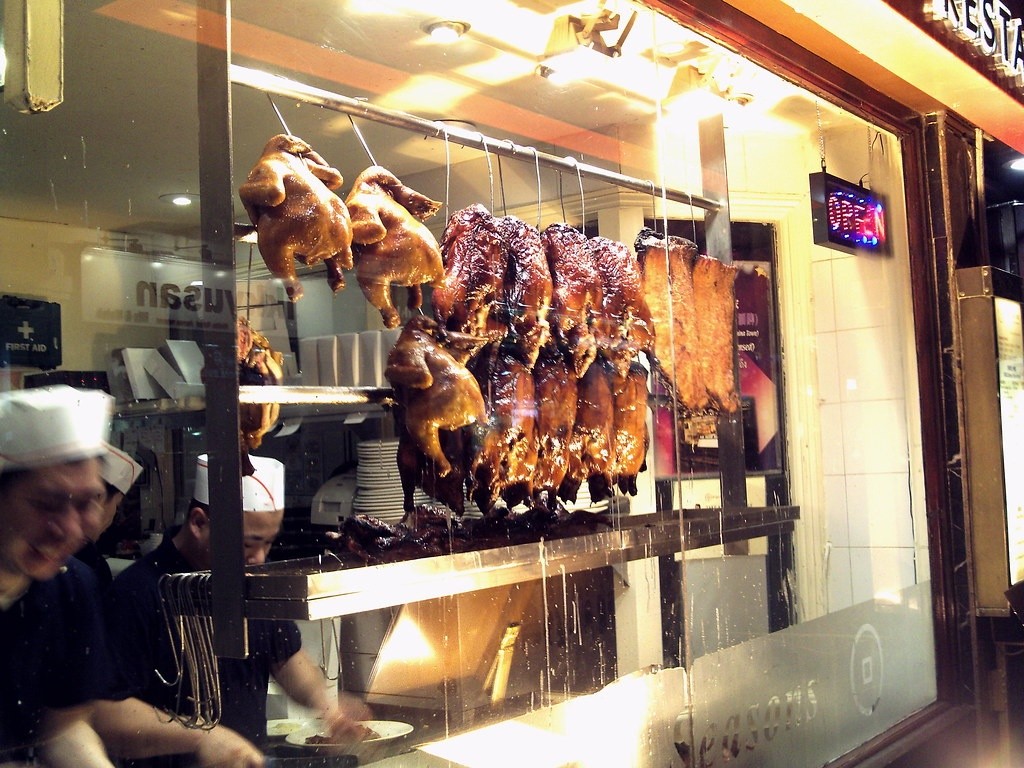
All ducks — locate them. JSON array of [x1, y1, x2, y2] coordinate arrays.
[[385, 202, 656, 515]]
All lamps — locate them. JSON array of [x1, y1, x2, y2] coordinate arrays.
[[661, 48, 759, 126], [539, 0, 637, 81]]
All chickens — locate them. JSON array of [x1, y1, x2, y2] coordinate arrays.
[[239, 134, 485, 476]]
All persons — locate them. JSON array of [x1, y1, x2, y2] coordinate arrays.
[[0, 386, 372, 768]]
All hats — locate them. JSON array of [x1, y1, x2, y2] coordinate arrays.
[[95, 442, 144, 496], [193, 451, 285, 514], [0, 385, 115, 473]]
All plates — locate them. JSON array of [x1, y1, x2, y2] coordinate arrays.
[[284, 720, 414, 763], [266, 718, 312, 737], [354, 439, 483, 521]]
